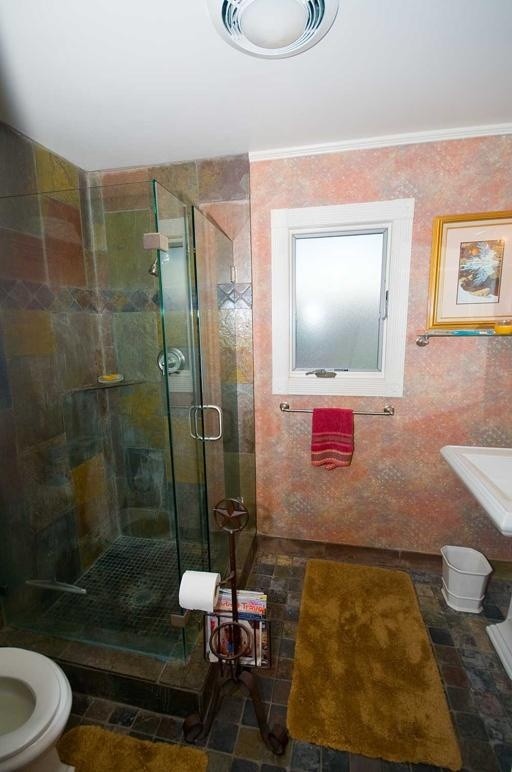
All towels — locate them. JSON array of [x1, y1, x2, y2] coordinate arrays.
[[308, 403, 359, 470]]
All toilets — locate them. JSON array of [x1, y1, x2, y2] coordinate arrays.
[[0, 646, 77, 772]]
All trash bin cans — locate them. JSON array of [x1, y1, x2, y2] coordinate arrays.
[[440, 545, 493, 614]]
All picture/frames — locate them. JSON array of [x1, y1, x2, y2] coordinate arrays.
[[426, 209, 512, 335]]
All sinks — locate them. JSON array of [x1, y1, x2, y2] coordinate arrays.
[[440, 444, 512, 538]]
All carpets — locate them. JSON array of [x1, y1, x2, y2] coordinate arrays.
[[282, 555, 466, 771], [55, 722, 210, 771]]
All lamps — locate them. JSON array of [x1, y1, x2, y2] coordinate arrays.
[[206, 0, 340, 63]]
